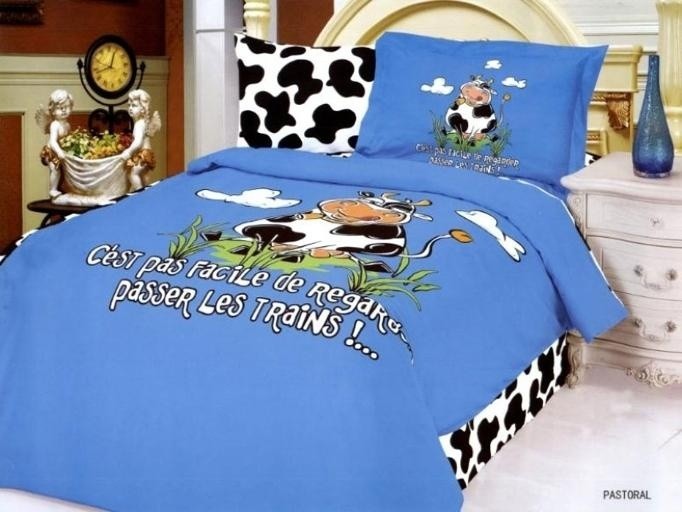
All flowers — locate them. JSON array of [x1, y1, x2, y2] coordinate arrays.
[[57, 127, 136, 159]]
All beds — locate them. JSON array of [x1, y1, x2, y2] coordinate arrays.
[[0, 1, 643, 512]]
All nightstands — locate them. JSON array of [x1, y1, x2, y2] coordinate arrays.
[[560, 151, 682, 387]]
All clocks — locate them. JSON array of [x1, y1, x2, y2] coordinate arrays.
[[76, 33, 145, 135]]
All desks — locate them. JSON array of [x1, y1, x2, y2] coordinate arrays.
[[25, 196, 124, 228]]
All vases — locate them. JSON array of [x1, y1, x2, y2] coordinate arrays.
[[35, 88, 162, 207]]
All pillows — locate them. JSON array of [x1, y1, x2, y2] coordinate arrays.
[[353, 31, 608, 194], [235, 30, 376, 156]]
